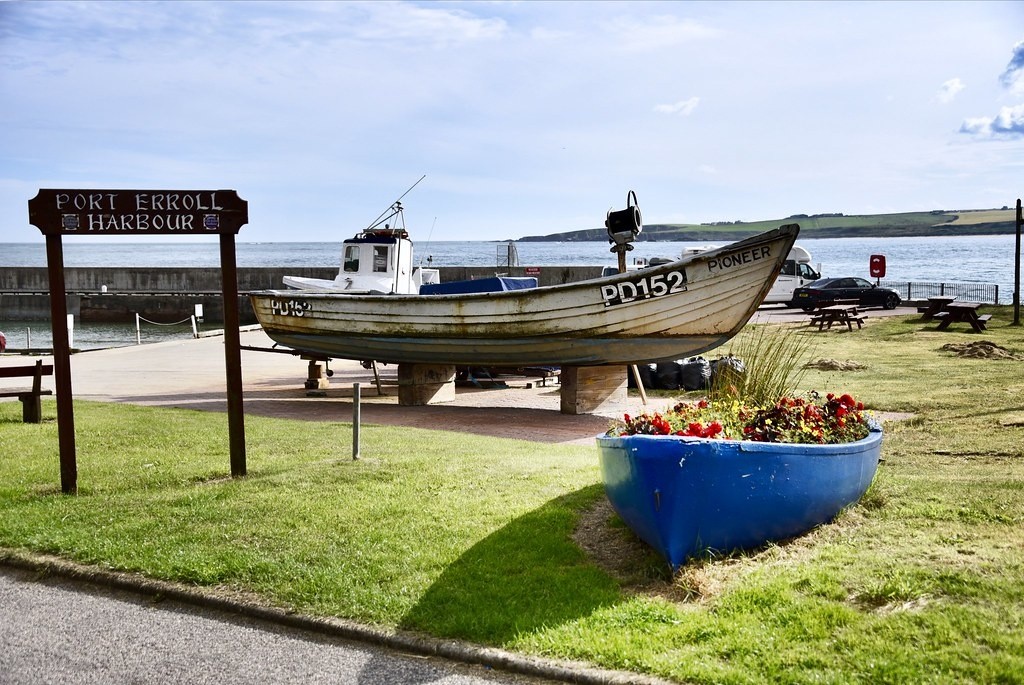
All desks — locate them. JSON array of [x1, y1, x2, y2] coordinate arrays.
[[919, 297, 957, 321], [935, 302, 987, 333], [819, 305, 862, 332]]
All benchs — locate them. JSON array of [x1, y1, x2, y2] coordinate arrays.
[[933, 312, 992, 333], [0, 360, 53, 423], [918, 305, 930, 321], [810, 315, 867, 332]]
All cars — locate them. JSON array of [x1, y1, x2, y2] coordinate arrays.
[[792, 277, 901, 311]]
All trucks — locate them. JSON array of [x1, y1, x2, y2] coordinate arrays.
[[681, 245, 821, 308]]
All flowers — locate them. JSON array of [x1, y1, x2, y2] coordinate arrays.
[[602, 310, 872, 445]]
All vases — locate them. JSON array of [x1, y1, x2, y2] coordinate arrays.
[[595, 432, 882, 575]]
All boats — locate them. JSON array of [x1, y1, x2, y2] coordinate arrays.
[[282, 175, 538, 295], [252, 189, 801, 365], [596, 403, 884, 577]]
